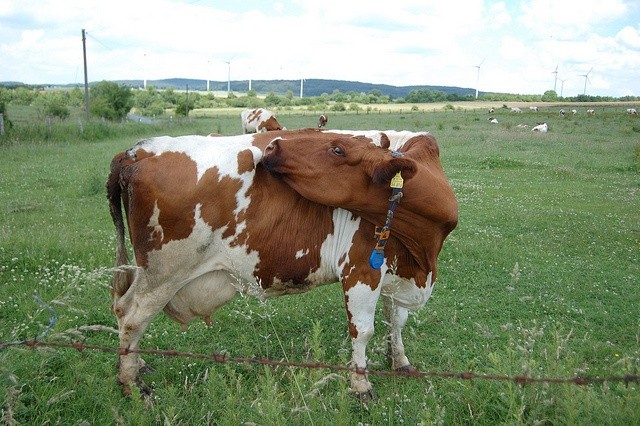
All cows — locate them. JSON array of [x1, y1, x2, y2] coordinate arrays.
[[585, 108, 596, 118], [488, 108, 495, 113], [517, 122, 530, 129], [488, 116, 499, 123], [529, 105, 539, 112], [531, 122, 550, 135], [241, 107, 287, 135], [106, 125, 460, 406], [559, 109, 566, 118], [318, 114, 329, 127], [571, 109, 577, 116], [625, 108, 638, 115]]
